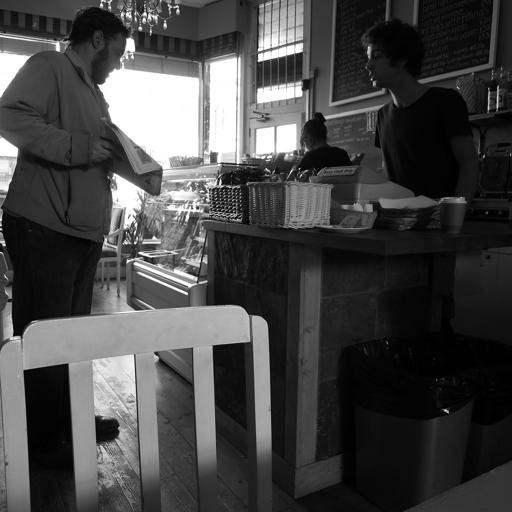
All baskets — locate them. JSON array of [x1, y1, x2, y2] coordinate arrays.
[[247, 181, 334, 230], [207, 184, 249, 224]]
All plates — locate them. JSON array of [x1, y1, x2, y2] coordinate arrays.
[[379, 196, 437, 233]]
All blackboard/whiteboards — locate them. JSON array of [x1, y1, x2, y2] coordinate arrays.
[[320, 105, 385, 184], [413, 0, 500, 85], [329, 0, 390, 108]]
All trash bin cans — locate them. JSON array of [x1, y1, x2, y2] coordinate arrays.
[[339, 333, 511, 512]]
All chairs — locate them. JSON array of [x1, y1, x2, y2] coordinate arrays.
[[0, 305, 273, 512], [96, 206, 126, 298]]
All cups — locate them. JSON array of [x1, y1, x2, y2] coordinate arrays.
[[440, 197, 466, 230]]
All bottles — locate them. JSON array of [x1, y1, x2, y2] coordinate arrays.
[[486, 67, 511, 112]]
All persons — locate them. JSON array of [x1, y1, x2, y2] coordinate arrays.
[[361, 18, 481, 211], [0, 6, 130, 471], [297, 113, 353, 173]]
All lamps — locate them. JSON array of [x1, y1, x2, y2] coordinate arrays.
[[97, 0, 180, 62]]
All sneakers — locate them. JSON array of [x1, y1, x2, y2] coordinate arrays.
[[95, 414, 119, 443]]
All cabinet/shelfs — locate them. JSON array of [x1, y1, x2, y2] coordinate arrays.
[[129, 164, 261, 388]]
